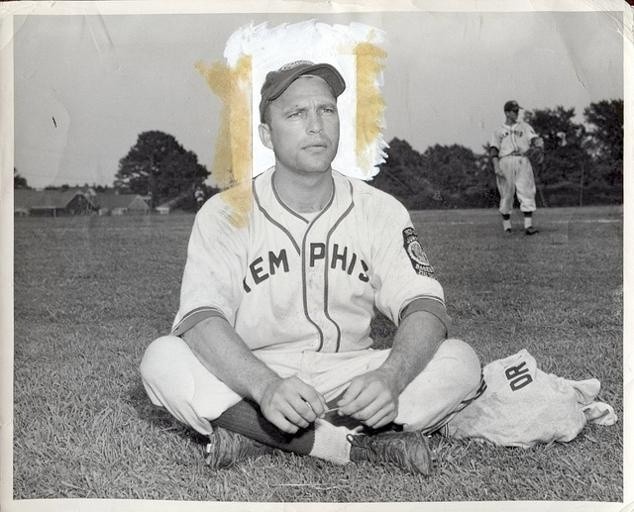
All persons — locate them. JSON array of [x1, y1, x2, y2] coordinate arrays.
[[489, 99, 545, 236], [137, 57, 483, 478]]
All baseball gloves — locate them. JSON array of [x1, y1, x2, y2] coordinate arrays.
[[529, 146, 544, 167]]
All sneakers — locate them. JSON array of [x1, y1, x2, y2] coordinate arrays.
[[350, 429, 432, 476], [206, 427, 274, 469], [525, 226, 540, 233], [504, 227, 513, 237]]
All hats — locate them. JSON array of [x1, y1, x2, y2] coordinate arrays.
[[260, 58, 345, 123], [504, 100, 524, 112]]
